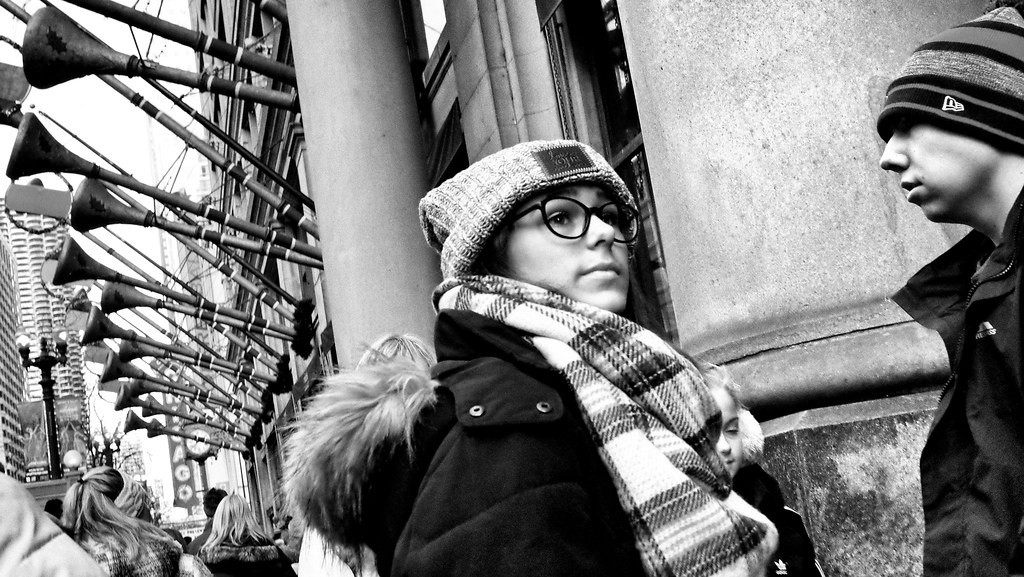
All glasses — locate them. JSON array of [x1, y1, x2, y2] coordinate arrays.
[[507, 195, 641, 243]]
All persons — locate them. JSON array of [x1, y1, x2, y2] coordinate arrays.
[[286, 139, 825, 577], [0, 467, 298, 577], [874, 7, 1024, 577]]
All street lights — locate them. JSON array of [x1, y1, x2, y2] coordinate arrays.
[[16, 325, 69, 479], [93, 436, 121, 467]]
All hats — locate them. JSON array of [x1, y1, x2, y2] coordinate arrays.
[[877, 8, 1024, 153], [416, 138, 641, 288], [203, 487, 228, 518]]
